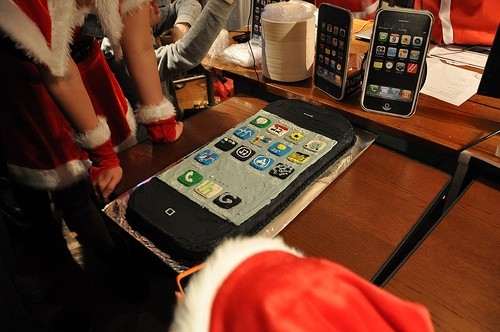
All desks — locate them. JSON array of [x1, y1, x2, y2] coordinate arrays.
[[377, 176, 500, 332], [202, 22, 500, 151], [108, 93, 454, 285]]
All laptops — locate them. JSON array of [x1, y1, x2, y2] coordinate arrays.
[[356, 28, 373, 42]]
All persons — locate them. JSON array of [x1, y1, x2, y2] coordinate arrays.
[[0, 0, 500, 332]]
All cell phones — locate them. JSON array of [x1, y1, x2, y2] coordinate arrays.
[[175, 262, 208, 299], [251, 0, 286, 46], [124, 98, 359, 270], [311, 3, 354, 102], [359, 7, 434, 119]]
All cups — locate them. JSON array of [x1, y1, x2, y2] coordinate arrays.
[[260, 11, 317, 82]]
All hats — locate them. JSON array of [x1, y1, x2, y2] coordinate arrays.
[[171, 231, 443, 332]]
